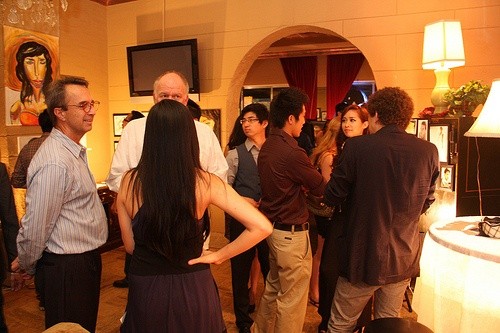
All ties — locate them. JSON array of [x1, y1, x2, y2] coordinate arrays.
[[240, 117, 259, 123]]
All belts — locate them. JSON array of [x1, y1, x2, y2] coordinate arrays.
[[43, 250, 99, 256], [272, 223, 309, 232]]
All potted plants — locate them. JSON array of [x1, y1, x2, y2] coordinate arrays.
[[443, 80, 492, 116]]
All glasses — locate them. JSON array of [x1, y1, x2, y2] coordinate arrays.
[[123, 117, 130, 123], [65, 100, 100, 112]]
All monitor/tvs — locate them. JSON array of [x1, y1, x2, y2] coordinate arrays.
[[126, 38, 199, 97]]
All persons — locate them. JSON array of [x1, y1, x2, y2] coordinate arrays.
[[324, 87, 440, 333], [12, 76, 108, 333], [0, 162, 20, 333], [224, 114, 260, 312], [250, 90, 326, 333], [113, 110, 145, 287], [296, 105, 374, 333], [107, 70, 229, 192], [117, 98, 274, 333], [224, 104, 271, 333], [14, 108, 54, 310]]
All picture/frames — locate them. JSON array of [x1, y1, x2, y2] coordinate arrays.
[[426, 124, 450, 164], [405, 118, 418, 136], [438, 164, 455, 193], [113, 113, 130, 137], [415, 119, 429, 141]]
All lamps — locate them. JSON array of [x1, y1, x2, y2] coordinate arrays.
[[422, 20, 466, 113], [464, 78, 500, 138]]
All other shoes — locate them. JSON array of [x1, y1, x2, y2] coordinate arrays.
[[310, 297, 319, 308], [248, 288, 255, 313], [24, 274, 36, 289], [114, 275, 128, 287]]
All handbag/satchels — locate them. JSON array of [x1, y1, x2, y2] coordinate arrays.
[[304, 149, 335, 218]]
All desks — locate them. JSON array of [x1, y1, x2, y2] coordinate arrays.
[[411, 216, 500, 333]]
